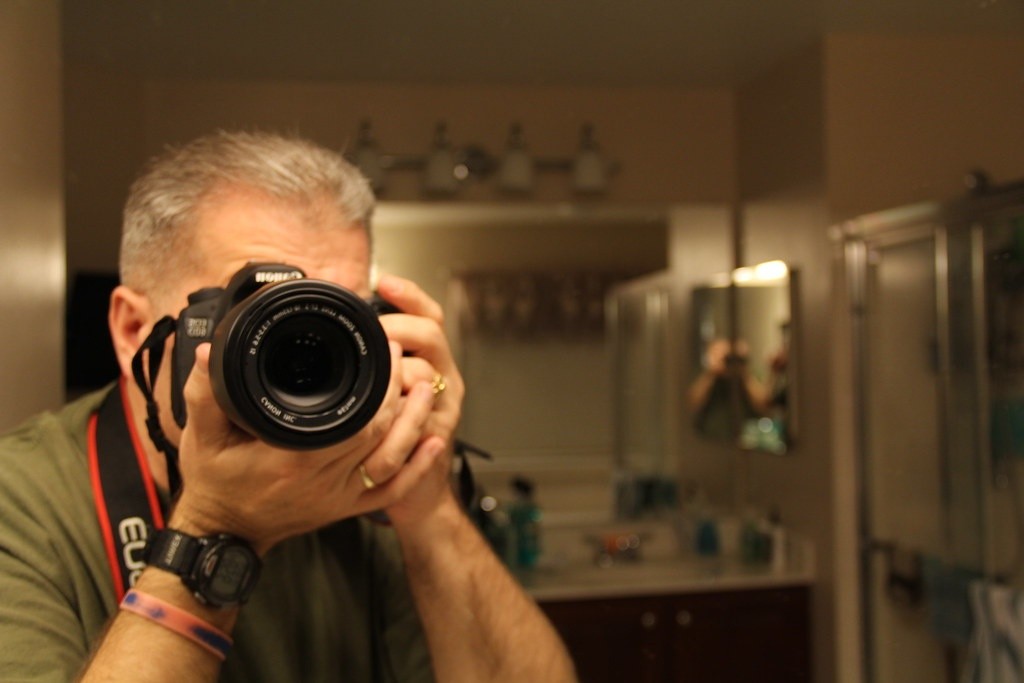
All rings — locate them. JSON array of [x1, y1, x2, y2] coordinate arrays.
[[431, 373, 445, 395], [360, 463, 376, 488]]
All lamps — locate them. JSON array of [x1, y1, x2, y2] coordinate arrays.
[[348, 115, 385, 195], [566, 121, 609, 194], [500, 123, 536, 198], [418, 121, 460, 196]]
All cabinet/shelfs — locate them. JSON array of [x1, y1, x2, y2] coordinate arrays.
[[534, 581, 816, 683]]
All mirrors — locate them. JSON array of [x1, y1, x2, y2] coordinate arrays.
[[685, 273, 732, 449], [728, 256, 802, 452], [369, 201, 741, 521]]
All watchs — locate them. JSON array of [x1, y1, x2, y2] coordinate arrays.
[[143, 526, 263, 608]]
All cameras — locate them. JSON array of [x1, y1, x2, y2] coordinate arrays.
[[171, 261, 416, 452]]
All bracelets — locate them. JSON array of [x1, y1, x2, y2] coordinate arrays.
[[120, 589, 233, 661]]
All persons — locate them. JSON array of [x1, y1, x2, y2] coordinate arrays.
[[0, 133, 577, 683], [687, 338, 765, 438]]
